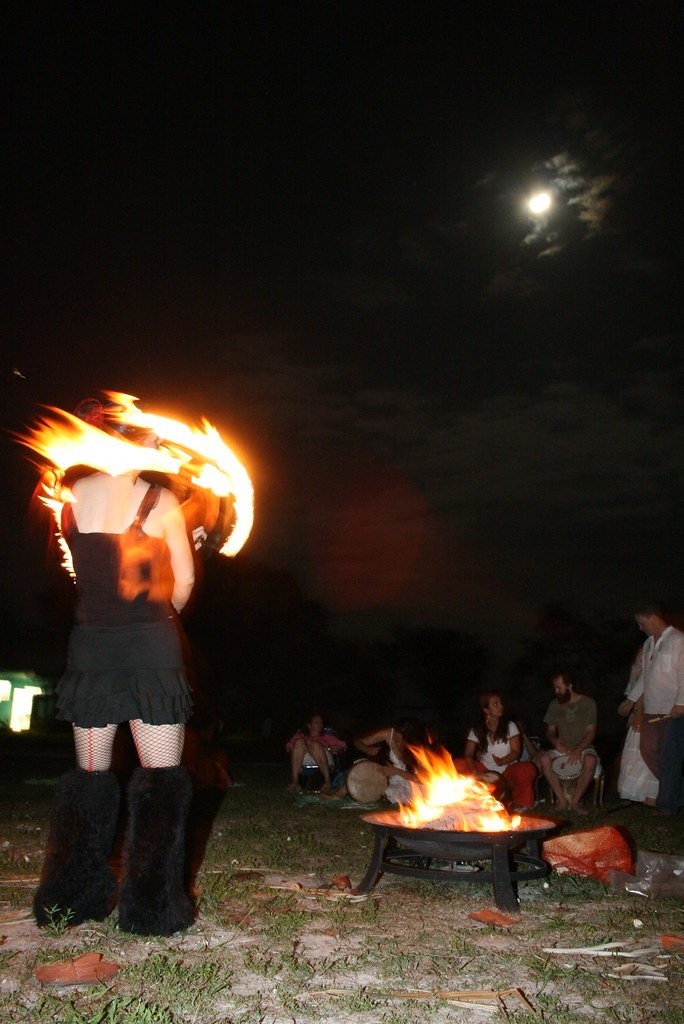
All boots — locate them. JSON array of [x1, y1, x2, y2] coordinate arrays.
[[120, 767, 195, 934], [34, 771, 121, 928]]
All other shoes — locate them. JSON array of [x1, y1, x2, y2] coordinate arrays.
[[571, 803, 588, 817], [548, 800, 568, 814], [642, 799, 657, 807], [321, 784, 331, 793], [650, 807, 672, 819], [284, 783, 301, 794], [319, 791, 345, 801]]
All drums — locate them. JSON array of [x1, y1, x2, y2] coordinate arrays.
[[551, 754, 586, 812], [472, 771, 512, 806], [344, 761, 389, 804]]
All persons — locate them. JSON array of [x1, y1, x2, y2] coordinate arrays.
[[180, 725, 229, 789], [464, 692, 521, 774], [540, 666, 597, 814], [616, 601, 684, 816], [285, 713, 346, 794], [33, 404, 198, 936], [514, 720, 539, 762], [320, 717, 420, 801]]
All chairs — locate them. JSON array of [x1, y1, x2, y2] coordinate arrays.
[[547, 762, 605, 808], [300, 728, 343, 785]]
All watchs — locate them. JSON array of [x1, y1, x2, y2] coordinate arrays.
[[327, 745, 330, 752]]
